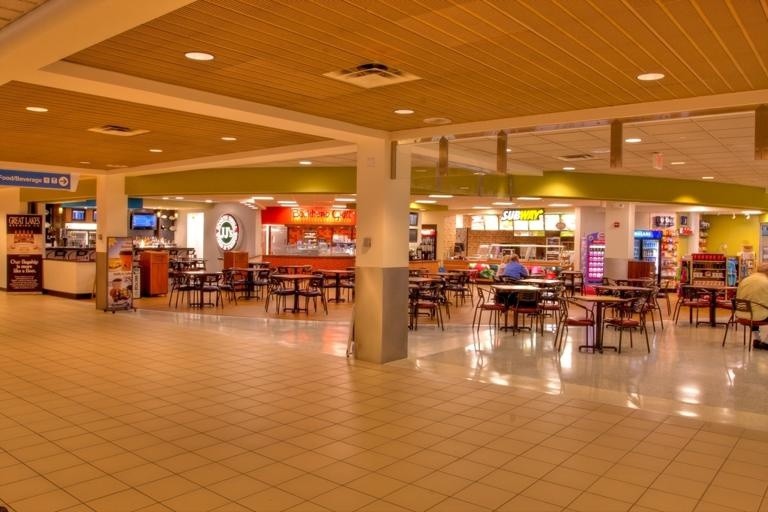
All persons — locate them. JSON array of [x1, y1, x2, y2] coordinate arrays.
[[735, 262, 768, 351], [446, 246, 470, 286], [495, 253, 540, 308]]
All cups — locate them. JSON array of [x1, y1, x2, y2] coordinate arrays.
[[119, 250, 132, 271], [297, 241, 302, 248]]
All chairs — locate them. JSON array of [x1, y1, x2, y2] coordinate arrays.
[[164, 245, 768, 360]]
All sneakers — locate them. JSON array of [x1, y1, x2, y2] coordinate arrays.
[[753, 340, 760, 349], [760, 342, 767, 350]]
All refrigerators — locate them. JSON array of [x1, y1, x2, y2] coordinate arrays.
[[57, 221, 97, 248], [421, 228, 437, 260], [633, 229, 663, 286]]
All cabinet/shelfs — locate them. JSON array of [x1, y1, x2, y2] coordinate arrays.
[[135, 249, 169, 300], [420, 223, 437, 260], [39, 246, 97, 302]]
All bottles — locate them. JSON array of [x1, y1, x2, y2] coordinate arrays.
[[422, 238, 433, 260], [348, 245, 357, 256], [336, 243, 349, 253], [68, 231, 96, 247], [308, 240, 312, 248], [318, 239, 328, 249], [635, 241, 657, 261]]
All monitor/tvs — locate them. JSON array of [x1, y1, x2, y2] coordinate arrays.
[[130, 212, 157, 230]]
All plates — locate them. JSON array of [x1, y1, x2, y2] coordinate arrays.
[[109, 257, 124, 271]]
